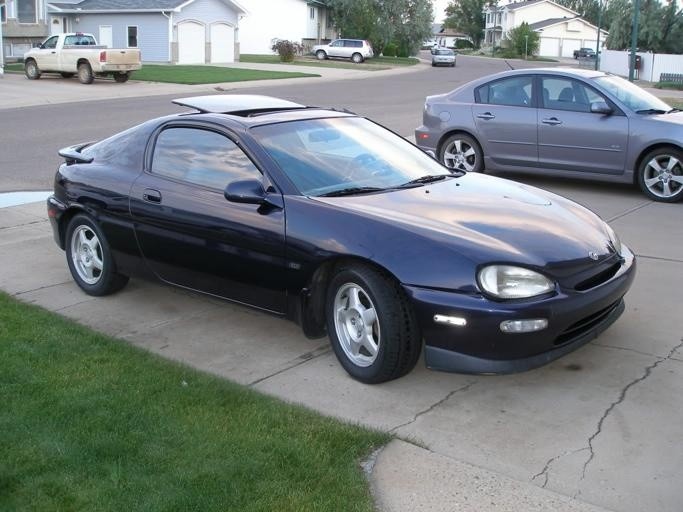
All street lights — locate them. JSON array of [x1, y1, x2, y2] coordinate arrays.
[[523, 32, 529, 60], [486, 1, 499, 58]]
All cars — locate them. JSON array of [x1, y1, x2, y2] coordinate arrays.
[[46, 95, 635, 384], [311, 39, 374, 63], [431, 49, 457, 67], [414, 67, 683, 203]]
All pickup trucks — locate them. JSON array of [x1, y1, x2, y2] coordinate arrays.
[[24, 32, 142, 84], [573, 48, 595, 59]]
